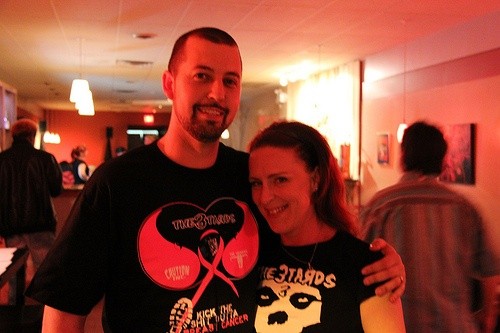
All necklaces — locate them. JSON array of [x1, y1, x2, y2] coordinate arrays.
[[281, 219, 324, 270]]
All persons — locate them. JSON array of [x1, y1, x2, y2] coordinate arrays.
[[362, 119, 499, 333], [250, 119, 406, 333], [59, 134, 158, 185], [25, 27, 406, 333], [0, 119, 65, 305]]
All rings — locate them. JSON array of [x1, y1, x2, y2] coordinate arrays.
[[400, 275, 404, 283]]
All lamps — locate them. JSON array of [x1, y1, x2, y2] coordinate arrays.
[[43, 111, 61, 144], [397, 44, 407, 144], [69, 38, 95, 116]]
